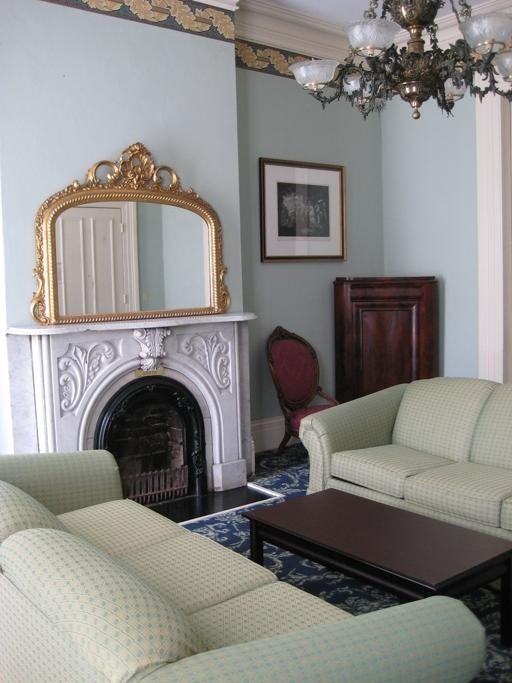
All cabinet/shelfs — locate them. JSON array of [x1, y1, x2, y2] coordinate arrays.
[[333, 275, 440, 403]]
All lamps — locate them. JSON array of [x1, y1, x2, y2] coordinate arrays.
[[287, 1, 512, 121]]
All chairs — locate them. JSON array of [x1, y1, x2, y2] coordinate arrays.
[[265, 326, 340, 456]]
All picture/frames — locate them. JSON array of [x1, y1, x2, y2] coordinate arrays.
[[258, 157, 347, 264]]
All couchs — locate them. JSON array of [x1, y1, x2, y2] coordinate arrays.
[[297, 377, 512, 595], [0, 448, 488, 683]]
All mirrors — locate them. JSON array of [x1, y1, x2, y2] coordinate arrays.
[[30, 140, 232, 327]]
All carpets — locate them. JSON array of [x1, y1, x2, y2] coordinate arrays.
[[179, 441, 512, 683]]
